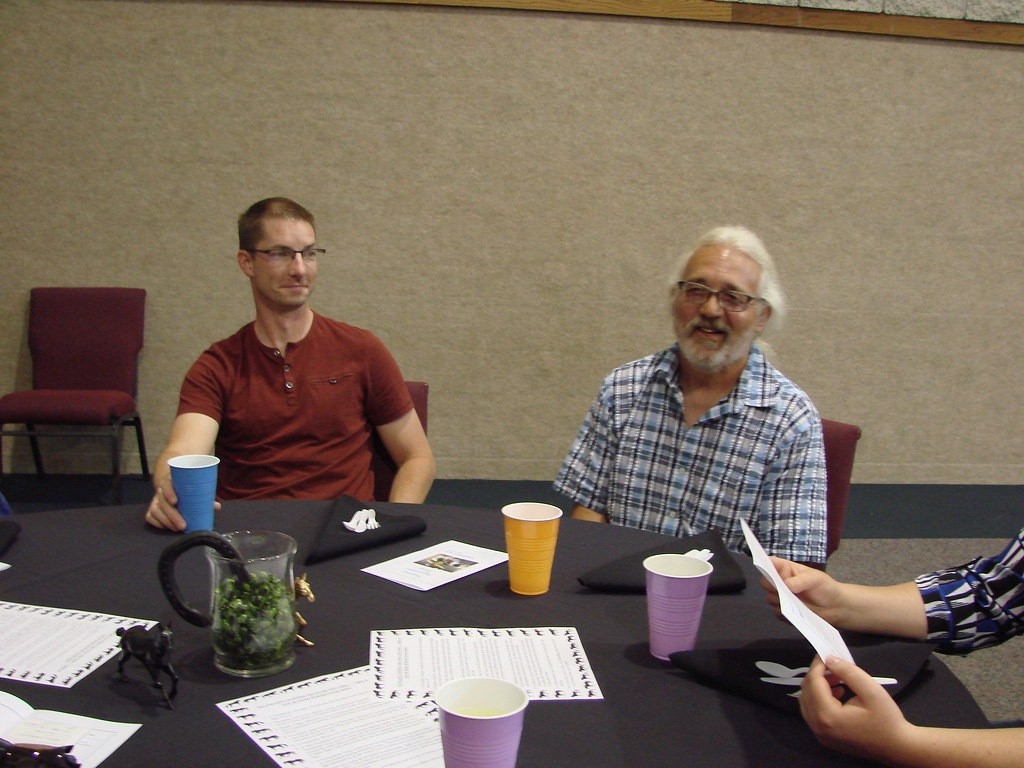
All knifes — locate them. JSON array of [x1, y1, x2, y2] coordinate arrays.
[[354, 509, 366, 533], [760, 677, 898, 685]]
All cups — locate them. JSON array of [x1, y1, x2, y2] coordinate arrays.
[[168, 454, 220, 533], [642, 553, 713, 661], [502, 502, 564, 595], [204, 530, 298, 678], [436, 676, 529, 768]]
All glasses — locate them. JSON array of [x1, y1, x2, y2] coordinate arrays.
[[247, 248, 327, 265], [677, 281, 767, 312]]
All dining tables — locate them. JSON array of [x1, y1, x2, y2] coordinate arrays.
[[0, 496, 988, 768]]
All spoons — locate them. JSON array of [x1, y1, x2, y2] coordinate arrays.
[[755, 661, 833, 677], [342, 511, 362, 531]]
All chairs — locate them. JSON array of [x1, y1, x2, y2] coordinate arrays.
[[822, 422, 864, 566], [0, 285, 150, 504], [363, 381, 428, 499]]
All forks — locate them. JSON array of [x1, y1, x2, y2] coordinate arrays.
[[366, 509, 381, 529]]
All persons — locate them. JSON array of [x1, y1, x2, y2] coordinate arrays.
[[146, 197, 435, 533], [757, 520, 1024, 768], [553, 227, 828, 573]]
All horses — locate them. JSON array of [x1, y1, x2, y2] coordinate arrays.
[[114, 621, 180, 711], [293, 571, 316, 648]]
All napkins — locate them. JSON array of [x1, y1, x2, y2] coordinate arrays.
[[582, 525, 751, 598], [308, 494, 428, 568], [666, 642, 937, 714]]
